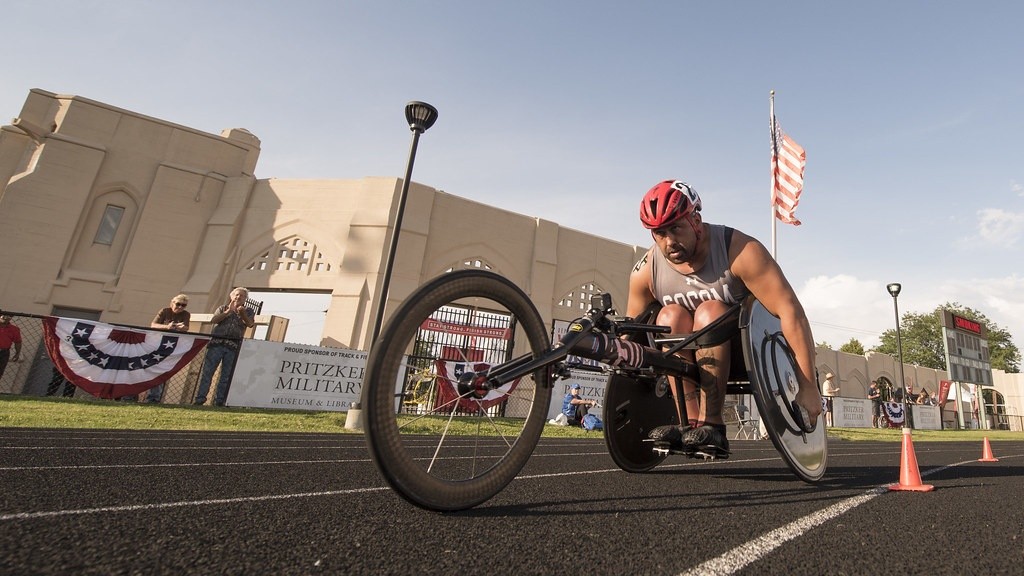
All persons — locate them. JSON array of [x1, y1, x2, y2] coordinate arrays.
[[620, 179, 822, 461], [868, 380, 882, 430], [562, 383, 598, 428], [823, 373, 840, 427], [122, 294, 190, 404], [193, 287, 254, 406], [0, 311, 22, 378], [903, 384, 940, 429]]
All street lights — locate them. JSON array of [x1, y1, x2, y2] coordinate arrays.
[[350, 100, 439, 409], [887, 283, 910, 428]]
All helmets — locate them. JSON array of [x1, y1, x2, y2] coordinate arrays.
[[639, 180, 702, 228]]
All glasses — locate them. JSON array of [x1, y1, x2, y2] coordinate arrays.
[[174, 302, 187, 307]]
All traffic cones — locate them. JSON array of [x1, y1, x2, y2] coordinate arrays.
[[888, 427, 934, 492], [978, 436, 999, 462]]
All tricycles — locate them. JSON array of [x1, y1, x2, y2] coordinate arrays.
[[363, 269, 828, 511]]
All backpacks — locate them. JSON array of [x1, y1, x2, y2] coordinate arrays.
[[581, 413, 603, 431]]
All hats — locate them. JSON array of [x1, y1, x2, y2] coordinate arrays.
[[825, 373, 835, 380], [572, 383, 581, 389]]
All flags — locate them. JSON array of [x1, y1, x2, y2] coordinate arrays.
[[768, 116, 806, 227]]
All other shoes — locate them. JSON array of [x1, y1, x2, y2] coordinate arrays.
[[682, 426, 730, 452], [193, 400, 203, 406], [214, 401, 224, 407], [648, 423, 691, 448], [148, 399, 160, 404]]
